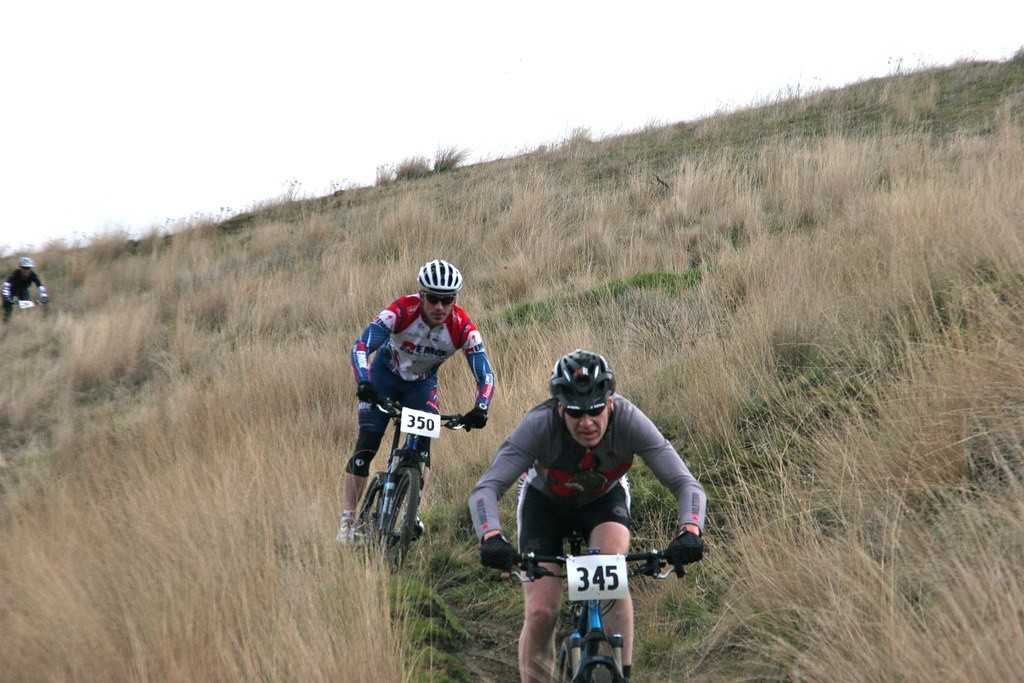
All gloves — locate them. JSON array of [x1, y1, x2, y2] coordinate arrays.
[[460, 407, 489, 432], [666, 527, 705, 578], [356, 381, 379, 404], [42, 297, 49, 303], [479, 532, 522, 573], [4, 296, 13, 306]]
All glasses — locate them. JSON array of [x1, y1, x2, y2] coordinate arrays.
[[19, 266, 32, 269], [562, 403, 607, 418], [423, 292, 455, 305]]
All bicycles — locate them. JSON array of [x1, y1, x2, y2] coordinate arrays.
[[344, 375, 489, 575], [6, 294, 49, 312], [489, 541, 705, 682]]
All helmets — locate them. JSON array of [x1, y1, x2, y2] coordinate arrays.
[[550, 349, 615, 408], [417, 259, 465, 294], [18, 257, 35, 268]]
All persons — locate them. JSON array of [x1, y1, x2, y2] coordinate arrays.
[[335, 259, 494, 544], [1, 256, 48, 327], [467, 348, 707, 683]]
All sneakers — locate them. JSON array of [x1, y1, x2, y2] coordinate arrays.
[[337, 518, 356, 544], [402, 513, 425, 534]]
